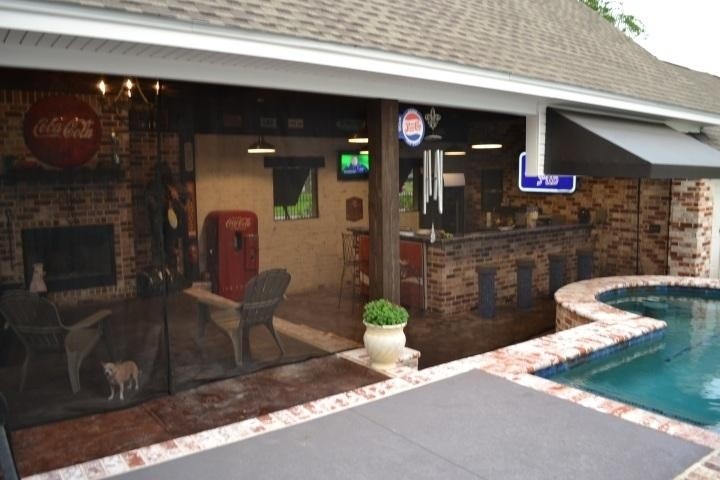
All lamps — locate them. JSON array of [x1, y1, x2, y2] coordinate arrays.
[[244, 134, 278, 154], [93, 74, 165, 115]]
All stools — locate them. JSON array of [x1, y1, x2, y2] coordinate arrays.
[[548, 253, 566, 300], [475, 264, 496, 318], [515, 259, 534, 311], [575, 247, 593, 281]]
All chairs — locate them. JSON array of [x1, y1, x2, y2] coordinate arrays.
[[1, 286, 122, 398], [335, 232, 365, 311], [198, 266, 291, 369]]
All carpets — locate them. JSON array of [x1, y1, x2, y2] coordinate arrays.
[[0, 289, 335, 431]]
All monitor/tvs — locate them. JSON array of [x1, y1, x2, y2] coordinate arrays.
[[337, 150, 372, 180]]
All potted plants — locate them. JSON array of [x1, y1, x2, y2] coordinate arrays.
[[359, 296, 410, 367]]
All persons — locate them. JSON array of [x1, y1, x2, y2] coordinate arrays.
[[144, 159, 180, 277]]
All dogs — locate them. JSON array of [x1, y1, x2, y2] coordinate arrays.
[[98, 358, 144, 402]]
